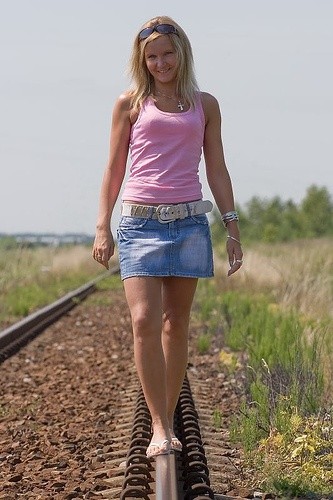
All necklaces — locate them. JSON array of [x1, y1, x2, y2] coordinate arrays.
[[153, 88, 184, 110]]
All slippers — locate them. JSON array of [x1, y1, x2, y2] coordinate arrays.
[[170, 438, 182, 451], [146, 440, 170, 458]]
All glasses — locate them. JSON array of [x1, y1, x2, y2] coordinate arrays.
[[138, 24, 180, 45]]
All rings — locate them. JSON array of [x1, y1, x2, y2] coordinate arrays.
[[236, 260, 242, 263]]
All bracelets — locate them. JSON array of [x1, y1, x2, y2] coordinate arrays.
[[222, 211, 239, 227], [227, 236, 241, 244]]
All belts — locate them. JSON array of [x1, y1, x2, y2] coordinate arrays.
[[122, 200, 213, 224]]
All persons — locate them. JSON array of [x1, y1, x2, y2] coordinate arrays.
[[92, 16, 243, 457]]
